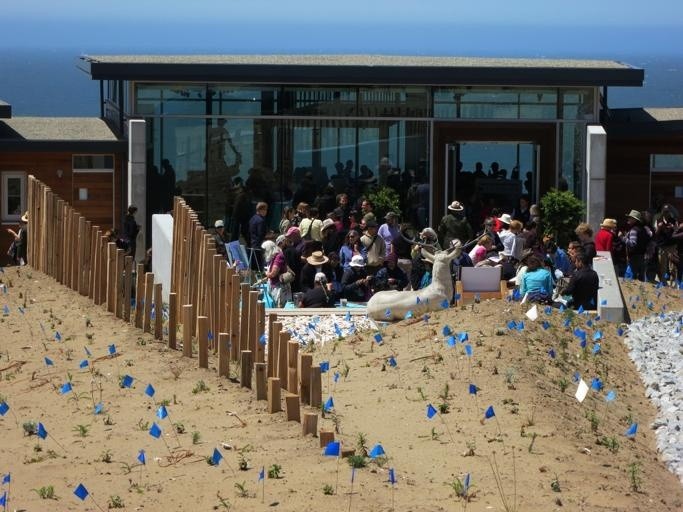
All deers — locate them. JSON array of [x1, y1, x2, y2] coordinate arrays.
[[366, 229, 489, 321]]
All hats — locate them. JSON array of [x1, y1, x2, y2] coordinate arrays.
[[349, 255, 366, 267], [307, 251, 329, 265], [321, 218, 337, 232], [286, 227, 299, 237], [315, 272, 327, 281], [364, 212, 378, 227], [497, 214, 511, 224], [215, 220, 224, 228], [626, 210, 641, 221], [448, 201, 463, 211], [600, 218, 617, 228], [384, 212, 400, 219]]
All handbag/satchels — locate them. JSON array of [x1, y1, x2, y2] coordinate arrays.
[[296, 235, 322, 257], [279, 272, 295, 284]]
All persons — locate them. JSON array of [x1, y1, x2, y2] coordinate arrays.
[[107, 205, 232, 297], [459, 161, 532, 233], [291, 162, 428, 229], [249, 193, 438, 310], [438, 201, 599, 310], [5, 211, 27, 266], [594, 209, 683, 291], [204, 118, 241, 159]]
